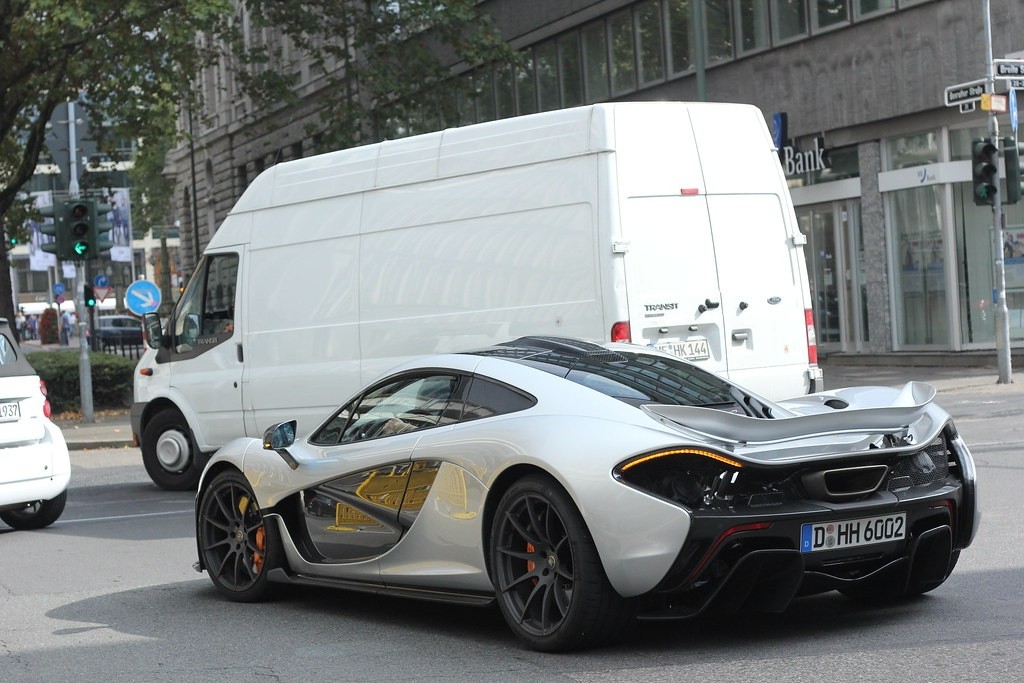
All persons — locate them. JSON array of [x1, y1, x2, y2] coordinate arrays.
[[14, 310, 41, 342], [110, 200, 130, 246], [60, 310, 81, 345]]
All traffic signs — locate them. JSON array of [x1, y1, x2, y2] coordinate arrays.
[[994, 57, 1024, 91], [945, 78, 989, 114]]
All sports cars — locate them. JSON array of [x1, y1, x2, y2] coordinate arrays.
[[194, 334, 986, 653]]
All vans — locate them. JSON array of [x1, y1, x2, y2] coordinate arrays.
[[132, 102, 822, 494]]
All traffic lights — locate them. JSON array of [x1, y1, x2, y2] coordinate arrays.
[[84, 283, 96, 307], [971, 136, 998, 207], [1003, 136, 1024, 206], [62, 198, 100, 262], [39, 196, 66, 261]]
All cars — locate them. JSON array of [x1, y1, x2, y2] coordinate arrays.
[[87, 313, 143, 352], [0, 317, 73, 536]]
